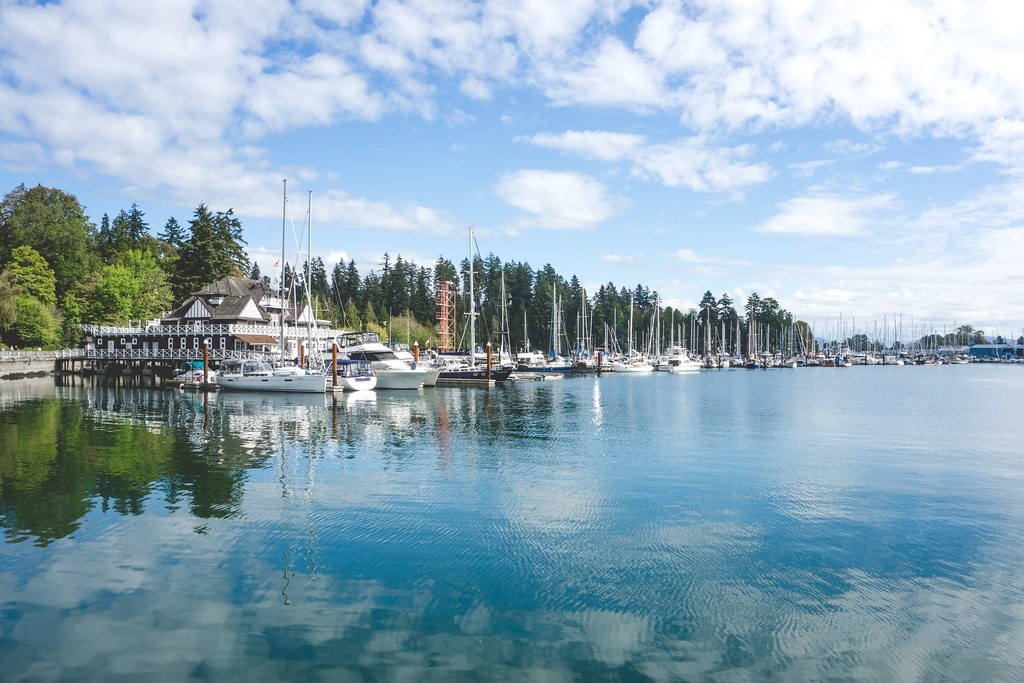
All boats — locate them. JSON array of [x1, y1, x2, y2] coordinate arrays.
[[329, 225, 1023, 389], [177, 362, 216, 386], [216, 179, 328, 392]]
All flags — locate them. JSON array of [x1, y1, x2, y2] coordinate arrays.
[[284, 309, 291, 320], [425, 338, 430, 349], [274, 260, 279, 267]]
